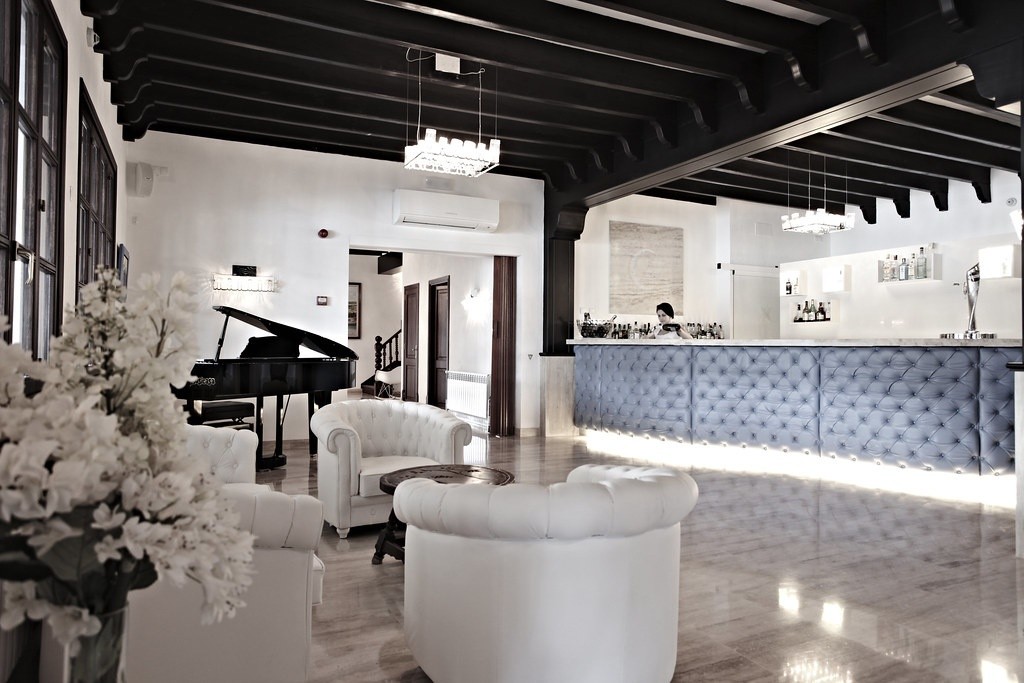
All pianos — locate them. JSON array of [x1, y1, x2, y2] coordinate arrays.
[[169, 306, 359, 472]]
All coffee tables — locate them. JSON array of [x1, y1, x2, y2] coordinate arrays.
[[373, 464, 514, 567]]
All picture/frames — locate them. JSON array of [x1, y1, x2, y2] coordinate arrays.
[[349, 282, 362, 339]]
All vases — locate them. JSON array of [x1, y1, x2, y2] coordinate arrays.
[[26, 602, 124, 683]]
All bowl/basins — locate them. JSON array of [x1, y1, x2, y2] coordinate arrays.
[[577, 319, 614, 337]]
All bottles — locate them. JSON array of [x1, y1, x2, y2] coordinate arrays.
[[816, 302, 825, 321], [581, 307, 662, 339], [808, 299, 817, 322], [792, 278, 800, 294], [682, 323, 724, 339], [785, 278, 791, 294], [794, 304, 803, 322], [654, 324, 681, 331], [802, 301, 810, 322], [826, 302, 831, 320], [881, 242, 936, 280]]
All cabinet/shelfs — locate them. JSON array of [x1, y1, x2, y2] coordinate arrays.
[[777, 243, 1024, 339]]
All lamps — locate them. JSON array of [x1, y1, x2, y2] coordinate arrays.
[[403, 48, 501, 178], [779, 151, 860, 236]]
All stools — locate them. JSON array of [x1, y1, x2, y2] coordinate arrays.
[[199, 400, 255, 430]]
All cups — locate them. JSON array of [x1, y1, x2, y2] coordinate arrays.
[[939, 330, 995, 339]]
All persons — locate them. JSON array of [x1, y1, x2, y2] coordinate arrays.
[[641, 302, 693, 339]]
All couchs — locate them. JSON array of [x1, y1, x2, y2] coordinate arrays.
[[394, 467, 696, 683], [308, 398, 473, 539], [117, 418, 326, 683]]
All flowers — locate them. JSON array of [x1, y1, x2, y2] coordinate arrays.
[[0, 260, 256, 657]]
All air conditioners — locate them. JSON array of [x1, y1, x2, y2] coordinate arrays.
[[392, 189, 499, 232]]
